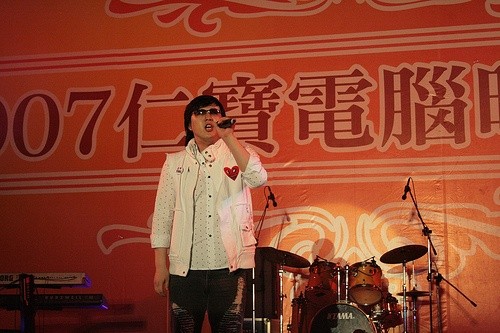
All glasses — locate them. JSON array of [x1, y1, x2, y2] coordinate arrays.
[[190, 108, 222, 116]]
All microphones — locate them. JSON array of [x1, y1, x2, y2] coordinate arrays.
[[217, 118, 236, 129], [268, 186, 277, 207], [402, 178, 410, 200]]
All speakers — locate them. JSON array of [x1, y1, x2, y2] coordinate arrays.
[[34, 304, 147, 333]]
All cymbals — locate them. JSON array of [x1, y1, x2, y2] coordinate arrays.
[[397, 291, 433, 297], [257, 247, 310, 269], [379, 244, 429, 264]]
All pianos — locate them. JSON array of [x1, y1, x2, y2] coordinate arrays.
[[0, 269, 112, 333]]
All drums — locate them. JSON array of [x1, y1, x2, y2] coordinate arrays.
[[288, 296, 317, 333], [309, 302, 379, 333], [307, 259, 342, 303], [345, 261, 383, 306]]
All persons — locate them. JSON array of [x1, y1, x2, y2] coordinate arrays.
[[151, 95, 268, 333]]
